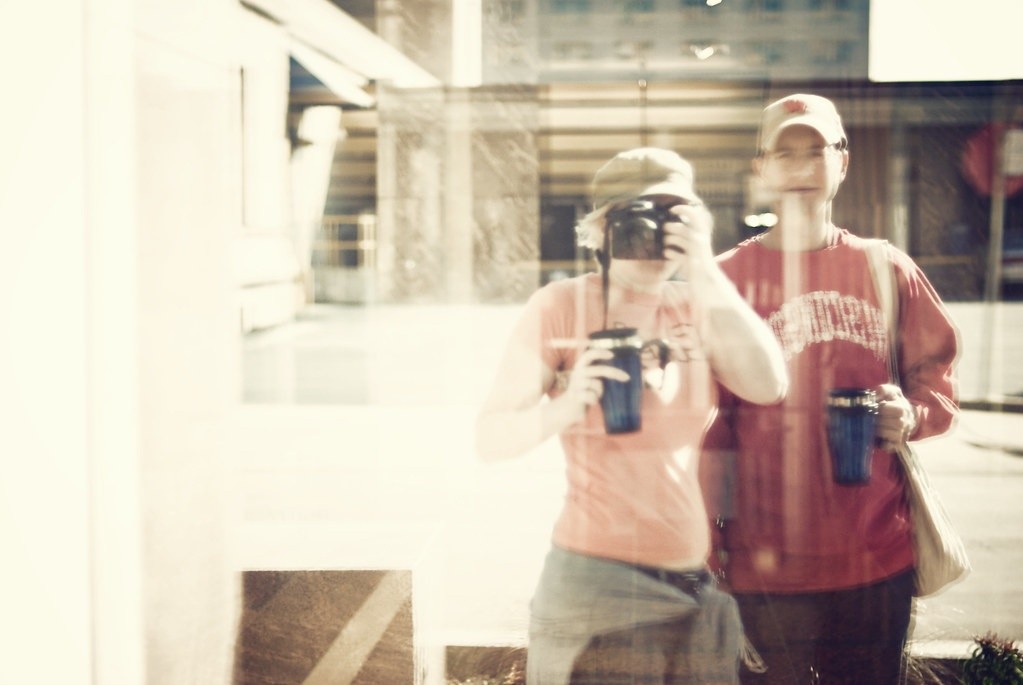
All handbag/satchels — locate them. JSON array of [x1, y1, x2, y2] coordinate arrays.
[[893, 442, 966, 597]]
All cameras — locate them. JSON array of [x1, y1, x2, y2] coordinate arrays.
[[608, 199, 685, 260]]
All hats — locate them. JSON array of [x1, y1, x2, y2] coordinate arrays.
[[758, 93, 848, 154], [591, 148, 701, 211]]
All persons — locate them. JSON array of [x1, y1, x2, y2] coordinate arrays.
[[485, 146, 789, 685], [696, 95, 960, 685]]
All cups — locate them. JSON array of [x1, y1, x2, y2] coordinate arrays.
[[825, 387, 887, 484], [587, 327, 643, 434]]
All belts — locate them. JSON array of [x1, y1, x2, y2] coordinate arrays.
[[641, 567, 713, 601]]
